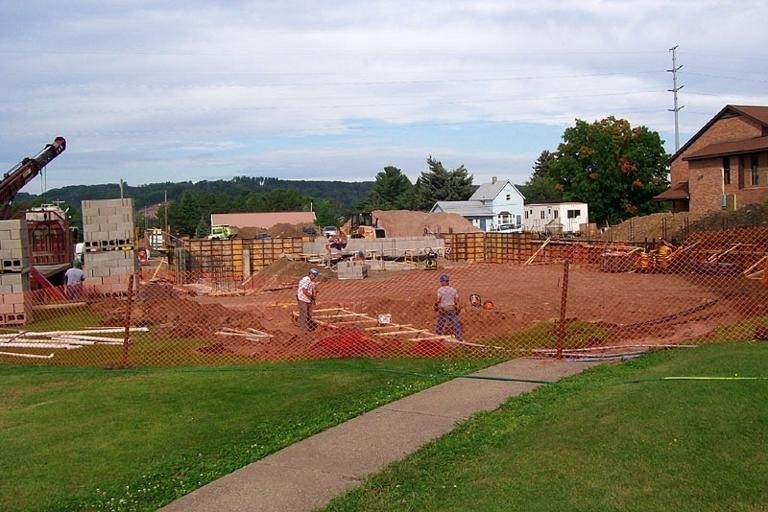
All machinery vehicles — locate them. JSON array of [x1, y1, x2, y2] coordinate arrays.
[[208, 224, 238, 241], [0, 137, 73, 290], [340, 212, 386, 238]]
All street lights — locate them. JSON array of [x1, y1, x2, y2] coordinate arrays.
[[120, 180, 127, 199]]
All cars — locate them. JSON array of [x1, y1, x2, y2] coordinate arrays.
[[496, 223, 522, 233], [255, 233, 272, 240], [322, 226, 339, 236]]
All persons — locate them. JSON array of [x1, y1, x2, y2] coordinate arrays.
[[432, 274, 462, 340], [296, 268, 320, 333], [62, 258, 84, 300]]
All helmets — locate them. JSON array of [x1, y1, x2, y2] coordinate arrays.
[[439, 275, 449, 281], [308, 267, 320, 277]]
